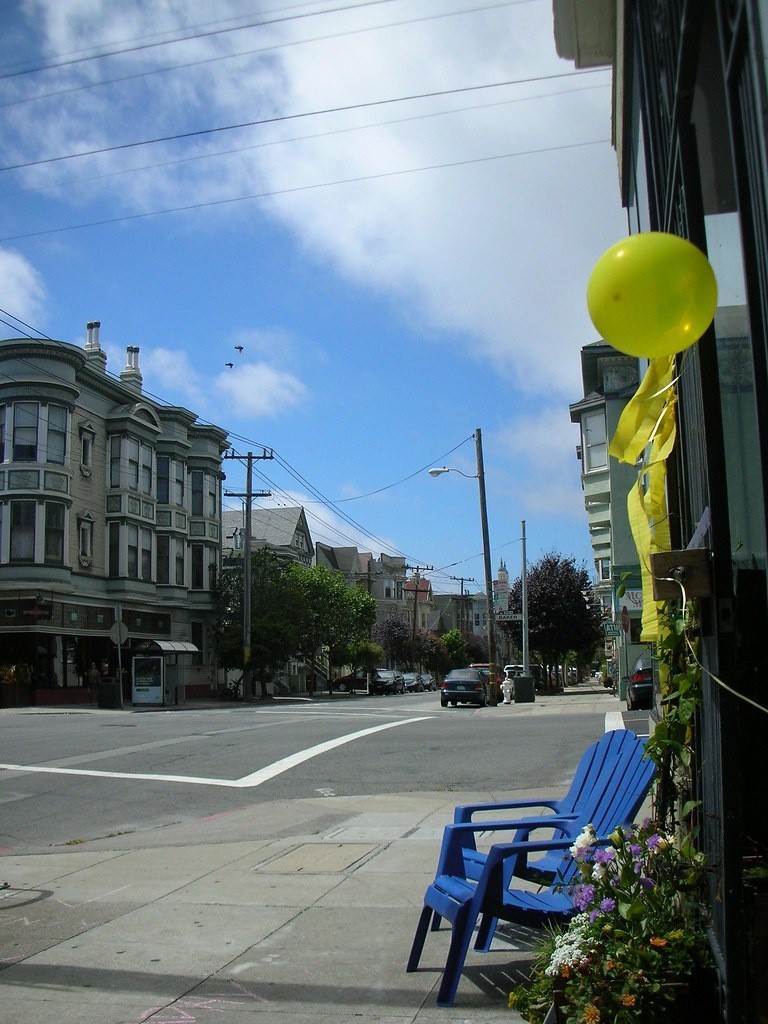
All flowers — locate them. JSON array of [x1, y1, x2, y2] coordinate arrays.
[[508, 512, 745, 1024]]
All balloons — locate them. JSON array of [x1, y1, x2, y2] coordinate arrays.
[[587, 231, 717, 360]]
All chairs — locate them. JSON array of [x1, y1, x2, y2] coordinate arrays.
[[406, 728, 659, 1007]]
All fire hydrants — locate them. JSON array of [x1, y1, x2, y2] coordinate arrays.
[[499, 678, 513, 704]]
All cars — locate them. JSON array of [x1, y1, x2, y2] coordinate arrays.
[[441, 669, 491, 707], [530, 664, 577, 681], [402, 672, 424, 693], [467, 663, 506, 705], [504, 664, 532, 677], [332, 668, 386, 692], [622, 650, 653, 710], [369, 671, 406, 696], [421, 674, 438, 691]]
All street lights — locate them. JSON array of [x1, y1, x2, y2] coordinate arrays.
[[429, 468, 497, 707]]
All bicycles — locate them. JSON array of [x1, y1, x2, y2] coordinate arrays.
[[222, 680, 243, 701]]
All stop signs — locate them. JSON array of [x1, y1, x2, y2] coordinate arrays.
[[621, 605, 629, 632]]
